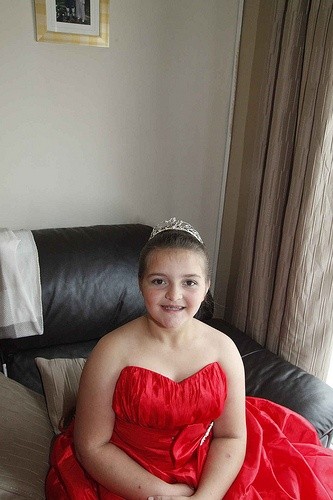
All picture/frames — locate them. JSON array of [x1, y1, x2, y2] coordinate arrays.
[[34, 0, 110, 47]]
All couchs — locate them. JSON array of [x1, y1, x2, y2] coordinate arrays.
[[0, 224, 333, 448]]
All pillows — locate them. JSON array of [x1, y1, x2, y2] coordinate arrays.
[[35, 357, 87, 434], [0, 372, 55, 500]]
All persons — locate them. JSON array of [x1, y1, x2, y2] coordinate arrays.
[[45, 217, 333, 500]]
